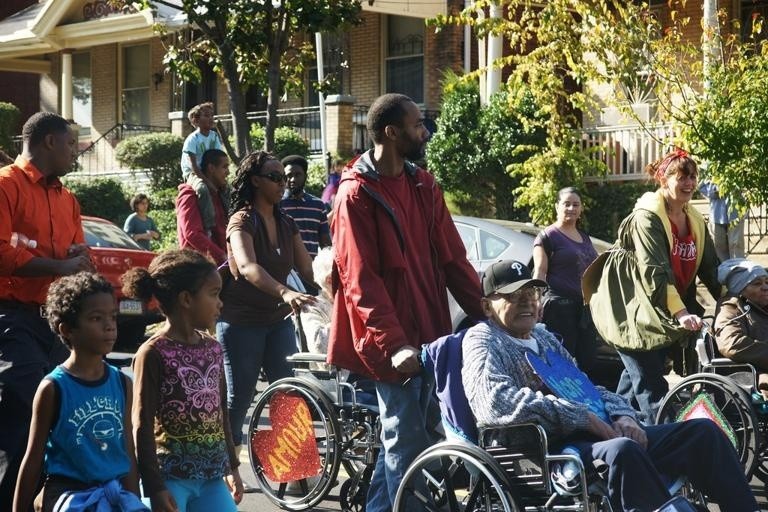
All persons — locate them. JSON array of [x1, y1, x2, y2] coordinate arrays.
[[118, 249, 244, 512], [459, 259, 759, 512], [713, 258, 768, 403], [12, 272, 152, 511], [300, 246, 378, 408], [696, 180, 747, 261], [321, 160, 345, 203], [176, 149, 229, 266], [175, 102, 231, 238], [123, 194, 162, 252], [0, 112, 95, 512], [279, 153, 333, 254], [215, 150, 322, 493], [616, 147, 729, 427], [326, 95, 489, 512], [532, 187, 599, 381]]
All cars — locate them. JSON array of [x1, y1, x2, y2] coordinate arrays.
[[80, 214, 166, 321], [448, 215, 613, 334]]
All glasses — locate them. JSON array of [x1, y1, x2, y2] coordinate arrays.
[[495, 288, 541, 301], [255, 172, 289, 183]]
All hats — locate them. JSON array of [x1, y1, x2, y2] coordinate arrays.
[[718, 258, 767, 293], [482, 259, 547, 297]]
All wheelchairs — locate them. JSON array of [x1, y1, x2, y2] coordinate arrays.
[[657, 316, 767, 484], [391, 329, 710, 512], [246, 302, 381, 511]]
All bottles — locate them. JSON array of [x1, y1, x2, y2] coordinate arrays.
[[10, 231, 38, 251]]
